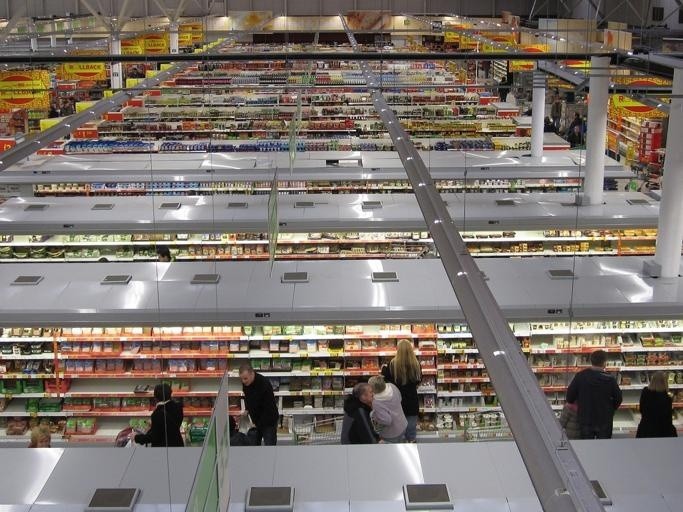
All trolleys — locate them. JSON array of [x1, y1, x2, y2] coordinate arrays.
[[464, 407, 516, 441], [294, 415, 344, 445]]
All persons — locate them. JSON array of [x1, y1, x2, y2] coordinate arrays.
[[237, 362, 279, 445], [340, 382, 384, 444], [27, 424, 52, 447], [367, 375, 409, 443], [381, 338, 423, 440], [559, 401, 579, 439], [636, 370, 678, 437], [566, 349, 623, 439], [544, 92, 587, 145], [127, 383, 186, 447], [498, 77, 509, 101], [157, 246, 176, 262], [229, 414, 263, 446], [50, 97, 80, 117]]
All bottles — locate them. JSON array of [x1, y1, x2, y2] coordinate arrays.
[[258, 107, 295, 121], [257, 142, 304, 152], [174, 70, 453, 86], [200, 180, 308, 196]]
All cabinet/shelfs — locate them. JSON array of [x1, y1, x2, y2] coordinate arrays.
[[0, 44, 683, 443]]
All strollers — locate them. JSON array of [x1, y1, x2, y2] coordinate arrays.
[[115, 421, 152, 447]]
